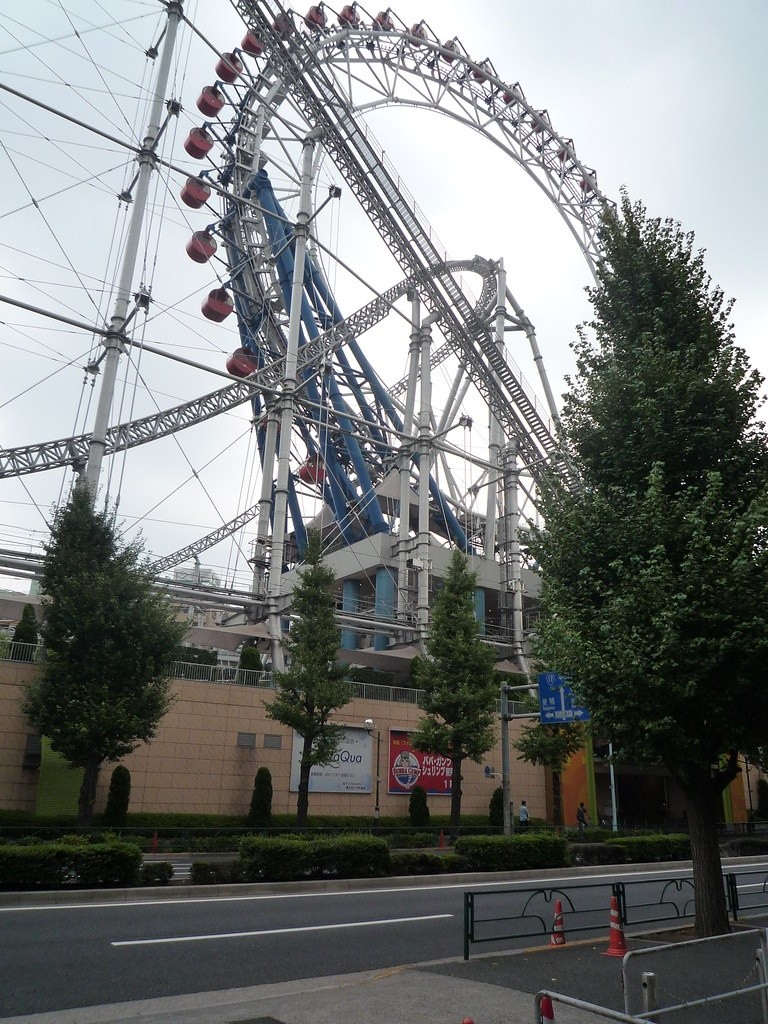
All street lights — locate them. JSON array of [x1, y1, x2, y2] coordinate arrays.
[[363, 718, 382, 828]]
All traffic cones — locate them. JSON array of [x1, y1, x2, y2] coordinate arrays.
[[152, 830, 159, 853], [547, 899, 567, 947], [437, 830, 448, 848], [540, 996, 557, 1024], [601, 897, 632, 956]]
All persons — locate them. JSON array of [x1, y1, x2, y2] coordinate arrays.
[[576, 802, 592, 830], [518, 800, 530, 827]]
